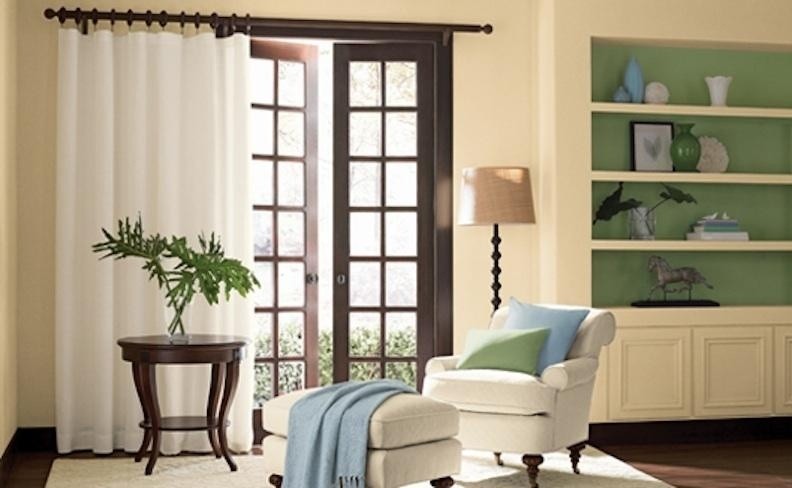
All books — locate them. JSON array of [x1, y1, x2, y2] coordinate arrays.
[[687, 219, 749, 241]]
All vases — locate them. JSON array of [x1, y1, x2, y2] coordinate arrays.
[[672, 121, 702, 171]]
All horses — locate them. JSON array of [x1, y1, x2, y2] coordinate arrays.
[[647, 255, 713, 302]]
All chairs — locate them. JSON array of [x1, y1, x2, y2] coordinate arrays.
[[422, 296, 618, 485]]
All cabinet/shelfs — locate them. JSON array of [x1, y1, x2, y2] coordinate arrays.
[[587, 34, 791, 423]]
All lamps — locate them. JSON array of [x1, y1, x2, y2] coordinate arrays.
[[458, 165, 539, 316]]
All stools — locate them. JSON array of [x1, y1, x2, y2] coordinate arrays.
[[254, 377, 462, 487]]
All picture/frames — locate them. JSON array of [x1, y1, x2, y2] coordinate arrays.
[[626, 118, 675, 173]]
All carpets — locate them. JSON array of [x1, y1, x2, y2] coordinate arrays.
[[41, 437, 680, 487]]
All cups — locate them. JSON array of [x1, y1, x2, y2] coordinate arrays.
[[629, 207, 655, 240]]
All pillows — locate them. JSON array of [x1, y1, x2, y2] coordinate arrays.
[[454, 302, 587, 378]]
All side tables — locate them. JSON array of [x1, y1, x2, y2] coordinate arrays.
[[115, 333, 246, 476]]
